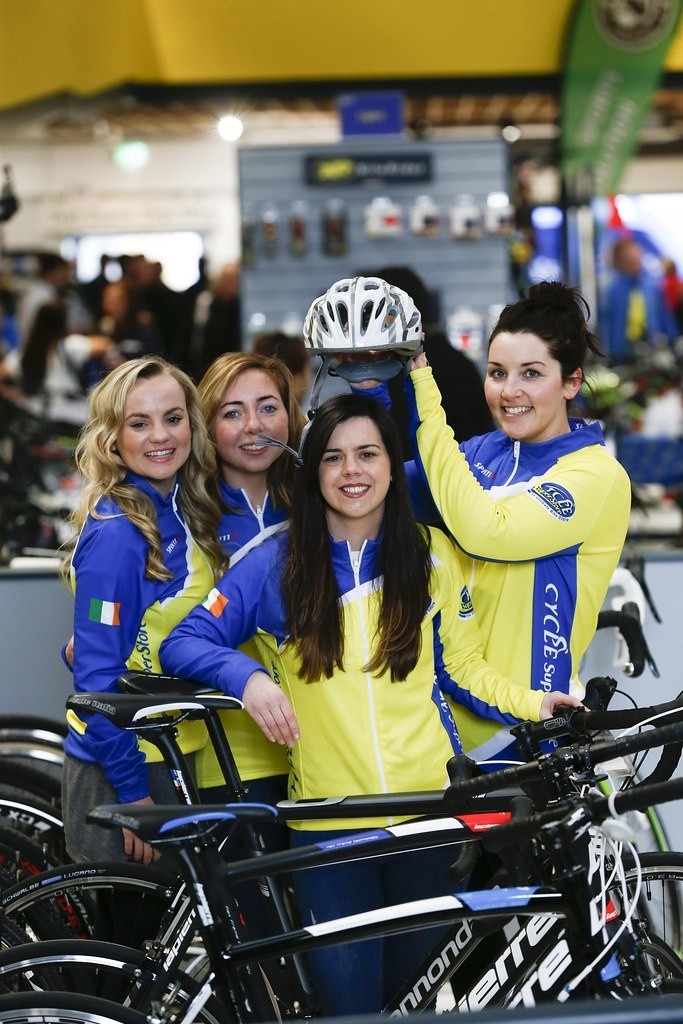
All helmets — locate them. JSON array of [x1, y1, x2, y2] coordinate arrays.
[[303, 277, 423, 382]]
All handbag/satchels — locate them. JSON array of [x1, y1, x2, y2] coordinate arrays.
[[60, 752, 196, 865]]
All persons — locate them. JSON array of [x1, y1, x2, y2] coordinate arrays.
[[60, 357, 231, 1013], [352, 266, 495, 463], [327, 283, 632, 774], [1, 160, 241, 398], [595, 239, 682, 370], [158, 392, 591, 1017], [61, 348, 309, 926]]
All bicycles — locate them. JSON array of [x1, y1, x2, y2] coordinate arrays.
[[0, 678, 683, 1024]]
